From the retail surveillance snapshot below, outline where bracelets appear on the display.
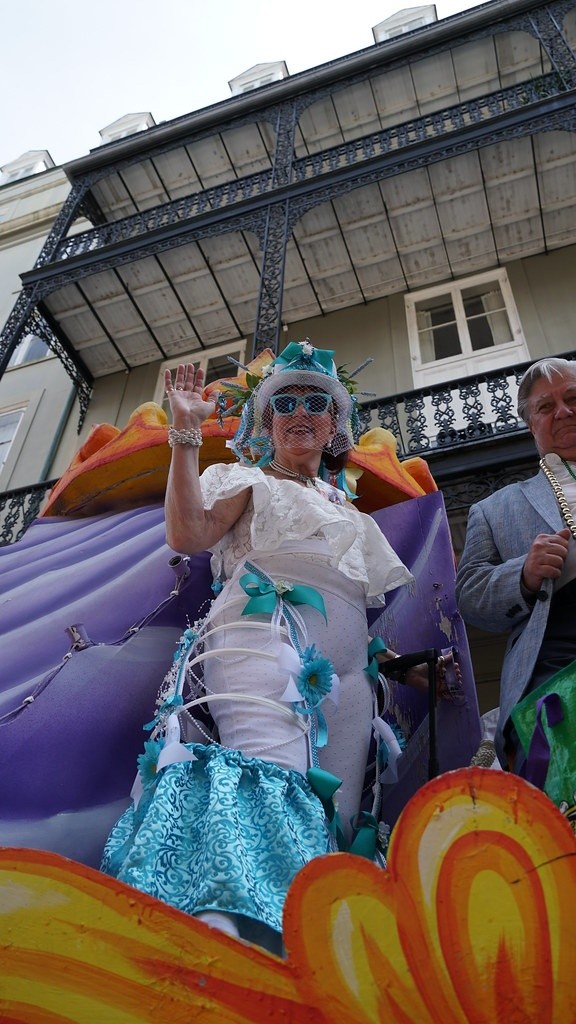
[390,669,408,685]
[168,427,203,447]
[395,655,401,658]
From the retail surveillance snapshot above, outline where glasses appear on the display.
[269,393,333,417]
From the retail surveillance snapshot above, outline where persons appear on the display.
[98,338,464,953]
[454,357,576,788]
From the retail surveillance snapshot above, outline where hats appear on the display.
[216,337,376,466]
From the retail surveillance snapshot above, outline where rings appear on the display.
[166,388,174,393]
[177,387,184,390]
[439,656,444,661]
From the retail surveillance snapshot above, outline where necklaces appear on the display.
[269,460,315,485]
[539,458,576,538]
[559,456,576,480]
